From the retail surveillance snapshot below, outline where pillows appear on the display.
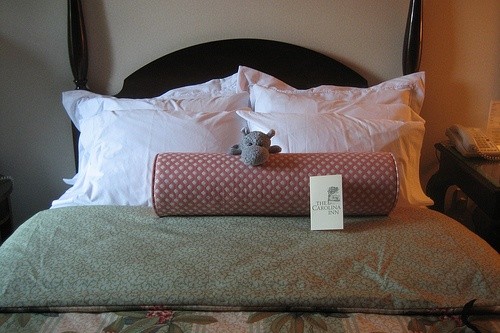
[240,64,426,116]
[153,151,401,218]
[239,107,436,212]
[54,107,247,206]
[61,74,250,188]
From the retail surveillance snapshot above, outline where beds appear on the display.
[0,1,499,333]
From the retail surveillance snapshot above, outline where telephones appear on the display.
[446,124,500,159]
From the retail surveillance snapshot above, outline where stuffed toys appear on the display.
[230,127,282,165]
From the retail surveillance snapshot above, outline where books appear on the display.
[309,174,344,230]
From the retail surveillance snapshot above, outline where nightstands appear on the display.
[426,139,500,252]
